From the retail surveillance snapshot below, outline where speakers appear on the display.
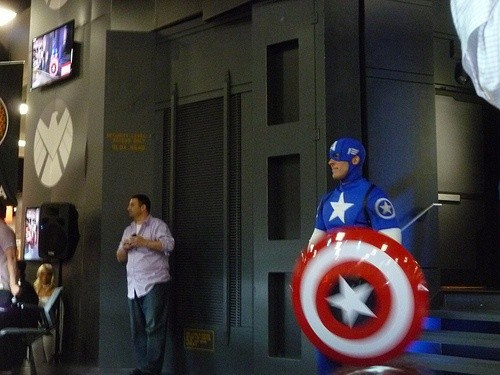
[38,201,79,262]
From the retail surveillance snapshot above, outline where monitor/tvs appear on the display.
[30,19,80,91]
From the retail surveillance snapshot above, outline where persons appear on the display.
[116,194,174,375]
[0,196,22,375]
[31,264,63,375]
[303,136,401,375]
[15,259,39,307]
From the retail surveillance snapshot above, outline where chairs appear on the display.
[0,287,63,375]
